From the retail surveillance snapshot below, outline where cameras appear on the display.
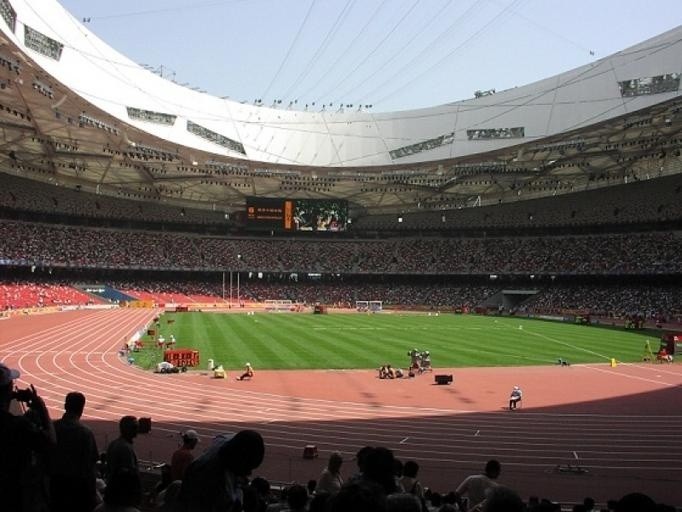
[15,389,31,402]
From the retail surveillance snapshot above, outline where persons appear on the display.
[0,232,682,326]
[1,171,246,226]
[0,331,682,512]
[292,200,346,231]
[350,172,681,232]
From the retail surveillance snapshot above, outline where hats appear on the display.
[0,364,19,385]
[183,430,200,441]
